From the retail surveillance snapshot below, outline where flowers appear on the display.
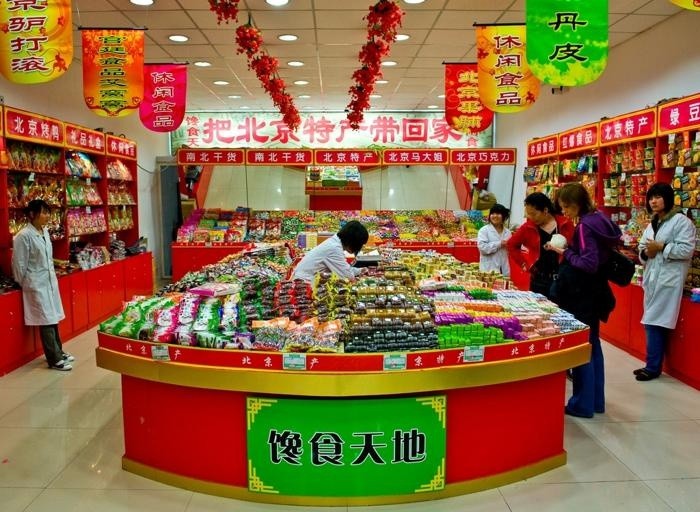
[350,64,384,94]
[264,73,287,96]
[251,48,279,77]
[362,0,406,44]
[279,102,302,133]
[235,12,264,55]
[347,110,364,131]
[271,89,294,106]
[208,0,240,25]
[348,85,369,102]
[346,99,370,113]
[358,35,390,73]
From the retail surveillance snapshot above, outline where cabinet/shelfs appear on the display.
[1,253,152,375]
[3,106,67,275]
[507,250,699,390]
[450,165,491,209]
[657,93,699,289]
[284,165,388,210]
[172,210,490,283]
[179,165,215,210]
[105,133,139,259]
[68,121,107,265]
[524,133,558,222]
[599,105,657,267]
[95,243,590,507]
[558,121,599,209]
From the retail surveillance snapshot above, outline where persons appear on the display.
[291,221,368,279]
[477,205,512,277]
[633,182,697,382]
[12,200,75,372]
[505,192,575,298]
[550,183,622,419]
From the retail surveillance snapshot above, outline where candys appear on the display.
[436,209,517,243]
[315,210,435,244]
[176,207,248,243]
[244,209,314,243]
[99,245,591,355]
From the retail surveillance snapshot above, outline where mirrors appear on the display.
[381,164,448,209]
[445,165,515,210]
[245,165,315,210]
[178,165,247,219]
[314,165,381,210]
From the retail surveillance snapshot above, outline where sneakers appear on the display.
[48,360,72,371]
[636,369,659,381]
[634,367,647,375]
[44,353,74,361]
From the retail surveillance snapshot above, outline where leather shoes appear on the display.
[565,405,593,418]
